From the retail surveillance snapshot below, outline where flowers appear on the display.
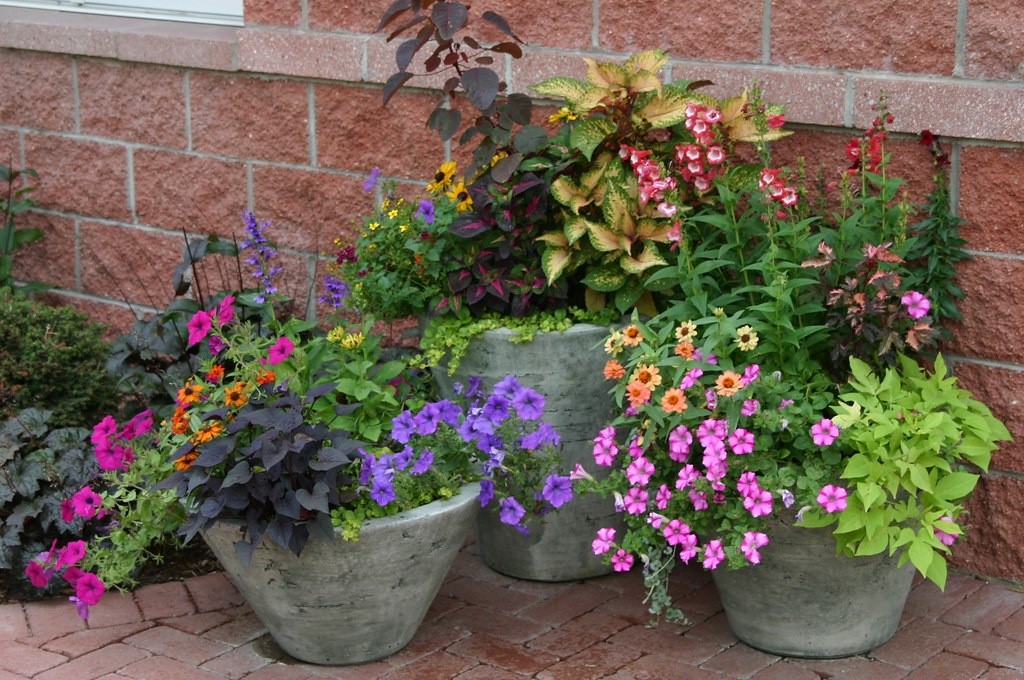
[313,0,786,380]
[28,213,572,630]
[567,83,1013,637]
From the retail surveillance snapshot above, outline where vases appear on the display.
[193,481,489,667]
[417,314,643,583]
[701,474,927,659]
